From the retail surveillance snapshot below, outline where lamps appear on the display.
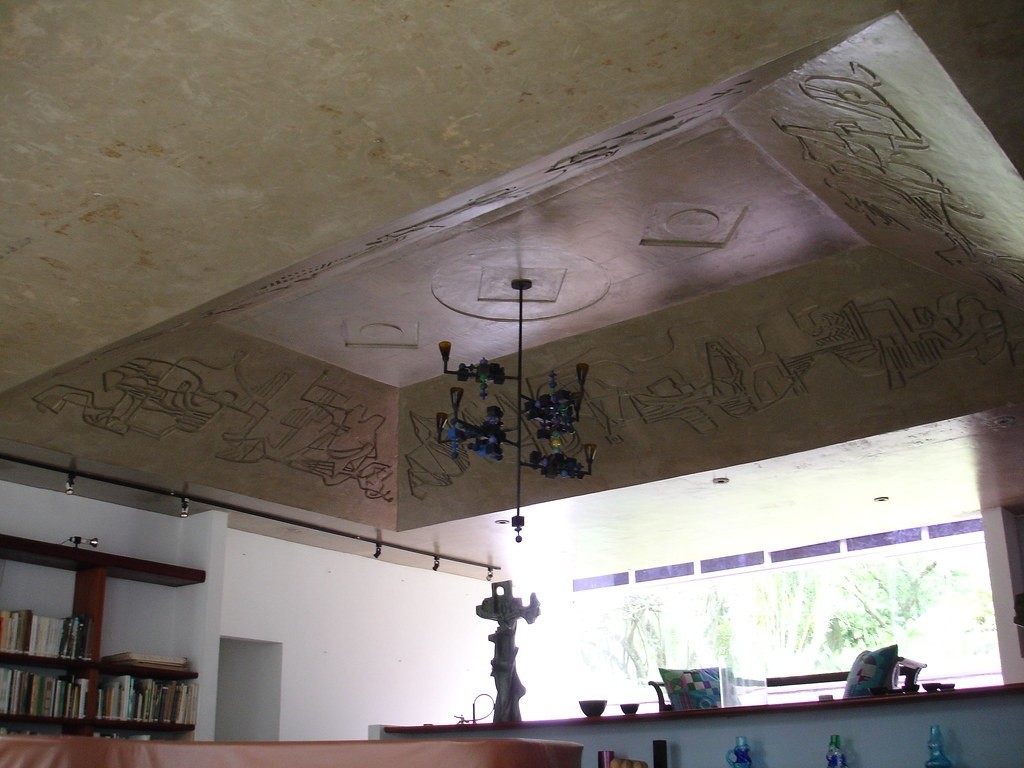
[65,474,75,494]
[486,567,494,581]
[435,279,598,545]
[68,536,99,547]
[432,555,439,570]
[373,543,382,558]
[181,496,189,517]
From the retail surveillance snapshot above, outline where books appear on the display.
[101,651,189,667]
[0,666,199,741]
[0,609,84,658]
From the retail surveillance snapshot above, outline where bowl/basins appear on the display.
[579,700,607,717]
[620,704,639,714]
[939,684,955,690]
[922,683,940,692]
[868,687,888,696]
[901,685,919,693]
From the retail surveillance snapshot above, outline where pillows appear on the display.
[659,667,721,712]
[843,644,899,698]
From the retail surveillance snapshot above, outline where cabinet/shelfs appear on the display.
[0,534,206,740]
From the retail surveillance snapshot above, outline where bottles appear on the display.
[725,736,752,768]
[924,725,953,768]
[826,735,848,768]
